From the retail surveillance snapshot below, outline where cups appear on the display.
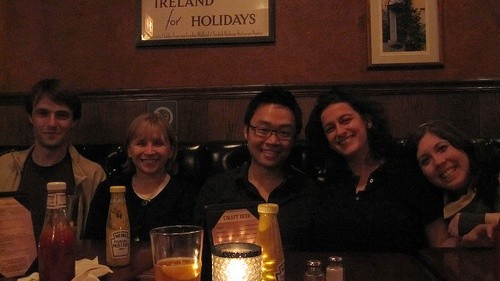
[211,241,263,281]
[149,225,204,281]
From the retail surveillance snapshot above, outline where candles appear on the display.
[211,242,262,281]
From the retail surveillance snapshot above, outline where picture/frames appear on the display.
[134,0,276,48]
[366,0,446,70]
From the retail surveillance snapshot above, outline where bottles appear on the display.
[37,181,76,281]
[326,255,345,281]
[254,203,286,281]
[303,259,325,281]
[105,185,131,267]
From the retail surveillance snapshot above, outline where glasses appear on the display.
[248,124,296,140]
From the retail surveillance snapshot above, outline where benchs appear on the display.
[0,137,500,249]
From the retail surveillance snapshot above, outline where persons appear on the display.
[402,120,500,248]
[192,89,324,252]
[304,86,428,251]
[82,112,196,242]
[0,78,106,240]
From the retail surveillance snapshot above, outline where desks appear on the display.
[35,239,500,281]
